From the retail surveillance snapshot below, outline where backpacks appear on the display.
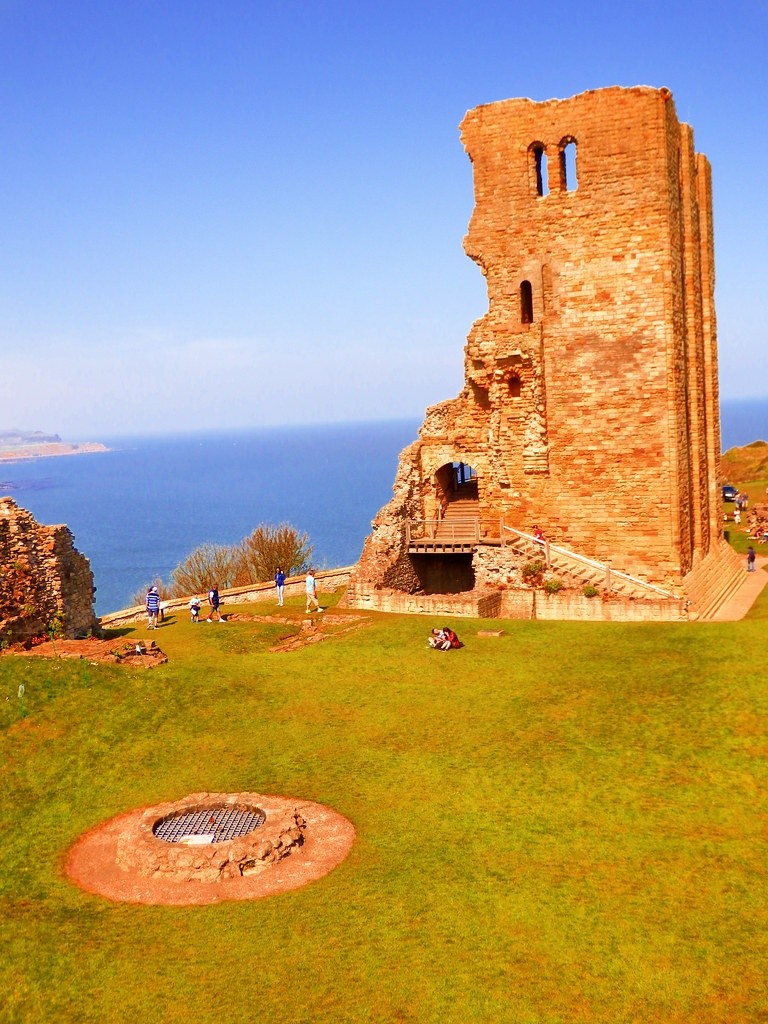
[208,590,213,605]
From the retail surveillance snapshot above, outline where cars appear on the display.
[722,485,740,502]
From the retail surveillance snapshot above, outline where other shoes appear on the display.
[306,609,311,613]
[317,608,323,612]
[438,648,448,652]
[196,621,201,623]
[277,603,283,606]
[219,619,226,622]
[191,617,194,622]
[207,619,212,622]
[147,623,151,630]
[154,626,159,630]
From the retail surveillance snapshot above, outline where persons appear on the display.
[734,490,748,511]
[206,584,226,622]
[305,570,323,613]
[146,586,160,630]
[532,525,543,556]
[758,531,768,544]
[428,627,460,653]
[747,546,754,572]
[734,507,741,528]
[274,567,286,606]
[757,525,764,538]
[190,592,201,623]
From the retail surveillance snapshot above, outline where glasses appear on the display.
[314,573,315,574]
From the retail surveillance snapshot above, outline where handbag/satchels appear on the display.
[435,640,446,649]
[750,558,754,562]
[191,605,197,615]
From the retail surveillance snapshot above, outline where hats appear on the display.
[153,587,157,591]
